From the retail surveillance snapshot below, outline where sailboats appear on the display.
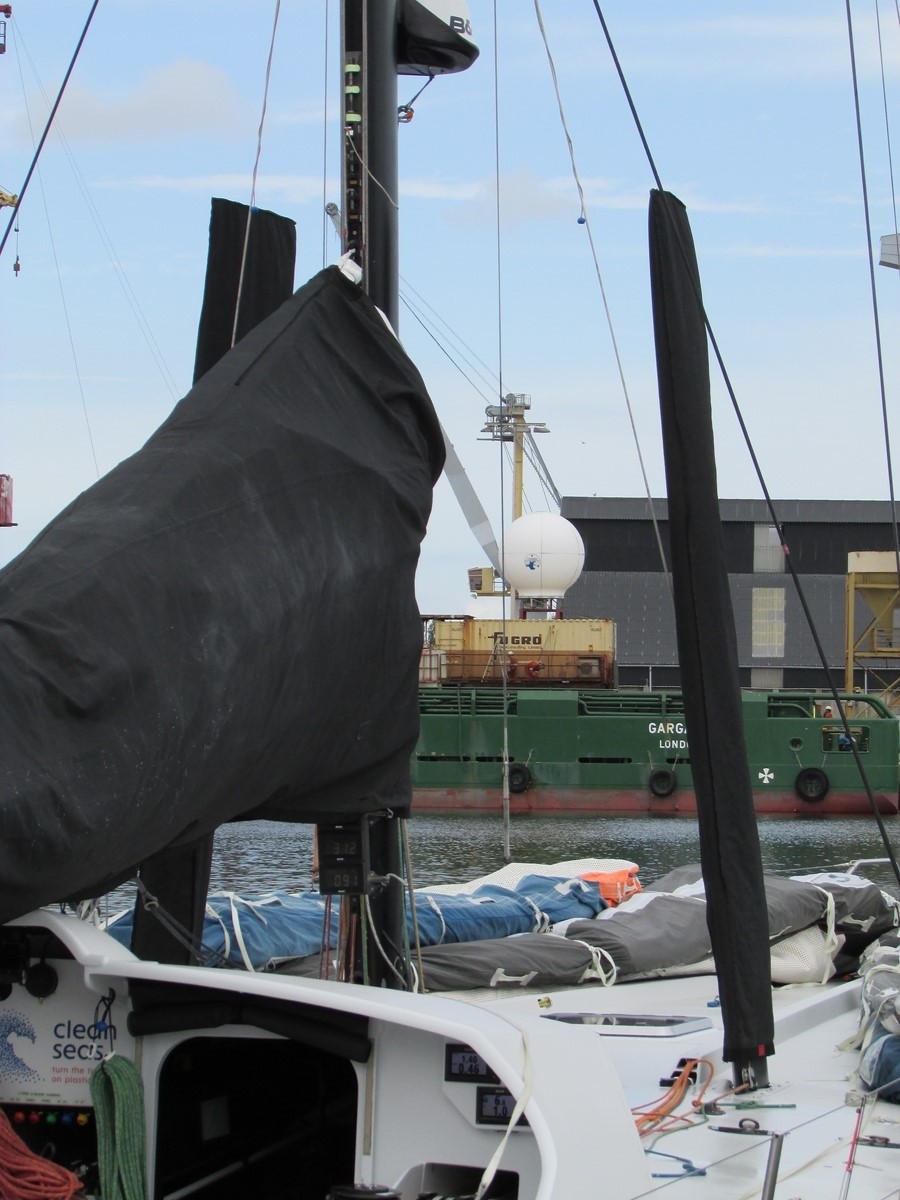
[1,1,900,1200]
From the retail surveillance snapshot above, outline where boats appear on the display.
[406,683,900,817]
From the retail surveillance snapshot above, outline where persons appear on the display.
[823,706,833,730]
[506,651,543,685]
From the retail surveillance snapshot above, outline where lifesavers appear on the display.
[648,768,676,796]
[503,763,531,793]
[795,768,829,802]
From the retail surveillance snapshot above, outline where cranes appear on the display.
[325,204,561,620]
